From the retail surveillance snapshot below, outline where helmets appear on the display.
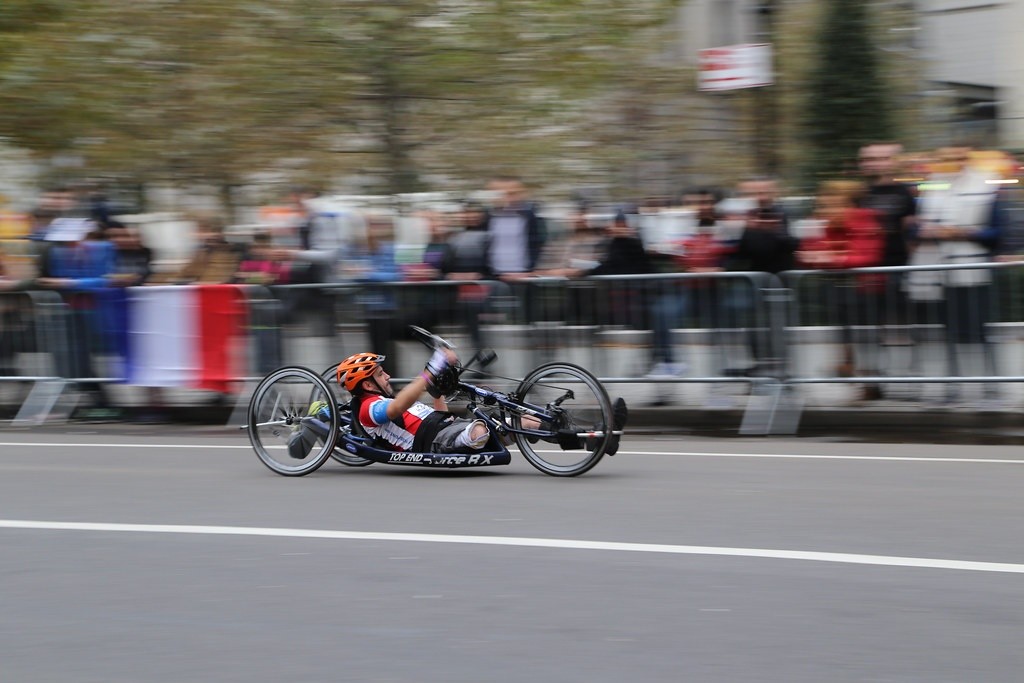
[336,352,386,395]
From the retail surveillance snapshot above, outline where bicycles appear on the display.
[246,324,615,478]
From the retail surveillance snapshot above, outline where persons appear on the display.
[338,348,559,455]
[0,144,1024,425]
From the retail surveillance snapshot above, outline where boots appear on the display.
[586,397,627,456]
